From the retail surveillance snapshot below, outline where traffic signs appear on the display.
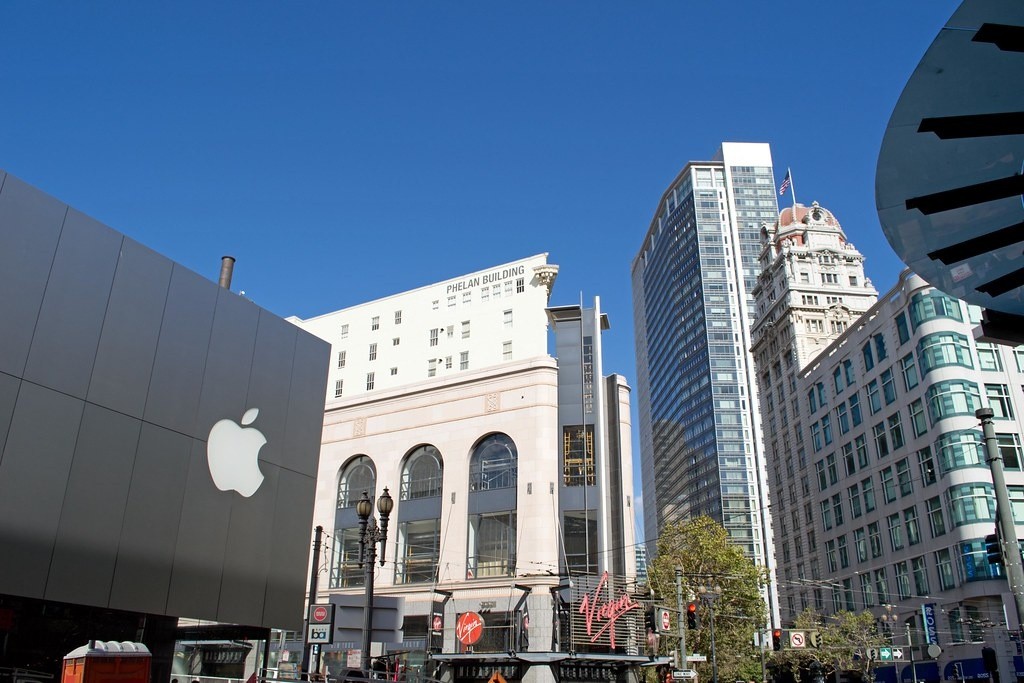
[672,669,698,680]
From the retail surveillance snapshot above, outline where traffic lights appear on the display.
[951,664,960,680]
[774,630,782,653]
[645,607,656,631]
[687,604,699,630]
[815,633,822,648]
[668,649,678,669]
[871,649,876,659]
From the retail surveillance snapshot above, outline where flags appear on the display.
[780,171,791,195]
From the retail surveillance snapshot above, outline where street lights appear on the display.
[698,577,722,683]
[355,485,394,679]
[882,607,900,683]
[909,646,920,683]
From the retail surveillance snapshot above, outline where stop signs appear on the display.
[660,612,671,631]
